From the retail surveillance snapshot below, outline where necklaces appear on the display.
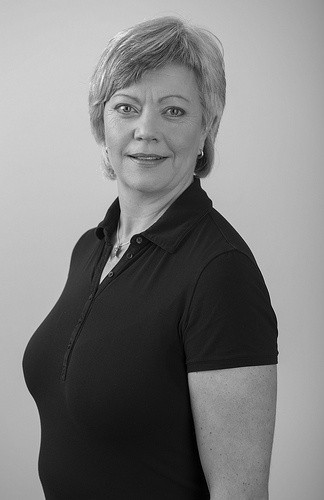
[114,219,130,256]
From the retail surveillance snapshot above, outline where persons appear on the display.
[22,16,280,500]
